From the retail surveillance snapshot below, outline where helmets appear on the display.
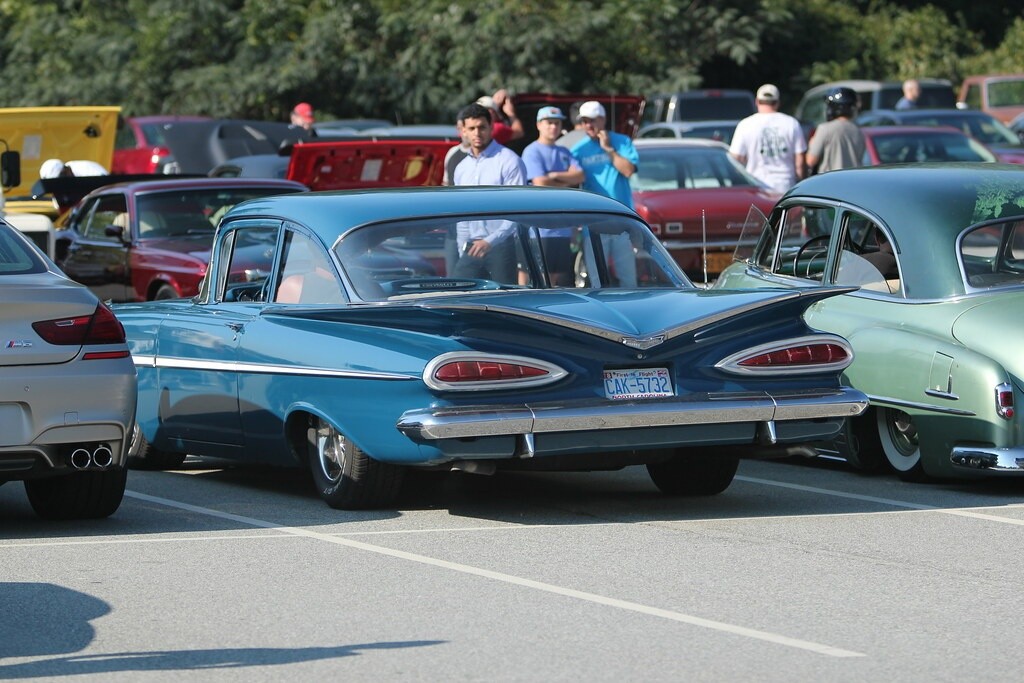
[821,87,857,104]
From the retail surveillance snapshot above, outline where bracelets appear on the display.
[607,146,614,153]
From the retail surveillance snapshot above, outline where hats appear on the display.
[293,102,316,123]
[756,83,781,102]
[536,106,568,122]
[38,157,64,180]
[474,95,507,122]
[575,101,607,121]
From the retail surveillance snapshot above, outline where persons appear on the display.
[520,106,586,290]
[290,100,318,140]
[473,91,524,145]
[554,100,640,288]
[895,79,920,109]
[870,221,894,255]
[804,86,865,176]
[727,84,807,198]
[441,103,527,286]
[39,158,111,218]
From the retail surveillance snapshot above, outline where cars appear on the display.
[51,176,439,308]
[708,158,1023,486]
[95,184,871,514]
[0,214,140,526]
[0,73,1024,282]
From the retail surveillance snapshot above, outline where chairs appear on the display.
[112,210,167,233]
[857,252,898,281]
